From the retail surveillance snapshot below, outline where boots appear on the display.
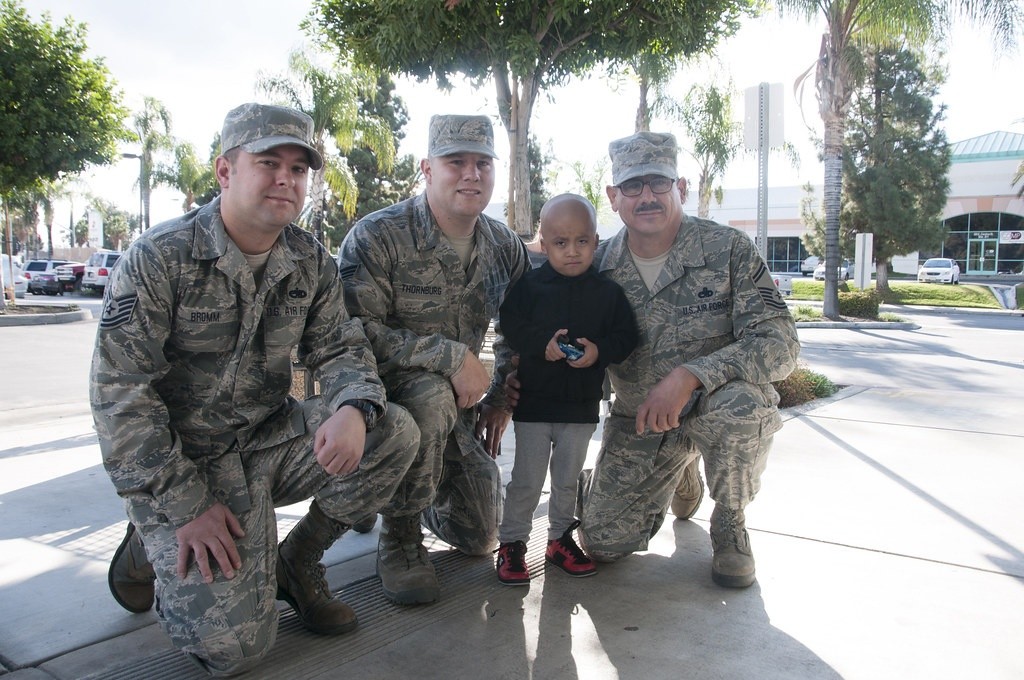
[375,509,442,606]
[108,520,158,613]
[273,501,357,632]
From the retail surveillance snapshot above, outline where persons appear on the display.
[503,131,801,587]
[336,114,534,608]
[492,193,639,585]
[88,102,422,680]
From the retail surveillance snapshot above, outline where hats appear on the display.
[220,103,322,171]
[609,131,678,185]
[429,115,498,160]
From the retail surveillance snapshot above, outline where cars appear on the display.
[813,258,854,281]
[31,273,58,296]
[801,255,821,276]
[918,258,960,284]
[2,254,29,297]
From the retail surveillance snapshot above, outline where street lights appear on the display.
[122,153,142,235]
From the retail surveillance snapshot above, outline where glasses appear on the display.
[615,176,680,197]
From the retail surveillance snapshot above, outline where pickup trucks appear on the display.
[771,275,792,298]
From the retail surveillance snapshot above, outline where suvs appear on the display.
[82,251,122,294]
[54,264,86,290]
[20,259,80,292]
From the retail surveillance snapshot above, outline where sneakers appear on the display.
[671,454,703,520]
[493,540,531,586]
[546,519,596,578]
[709,502,755,587]
